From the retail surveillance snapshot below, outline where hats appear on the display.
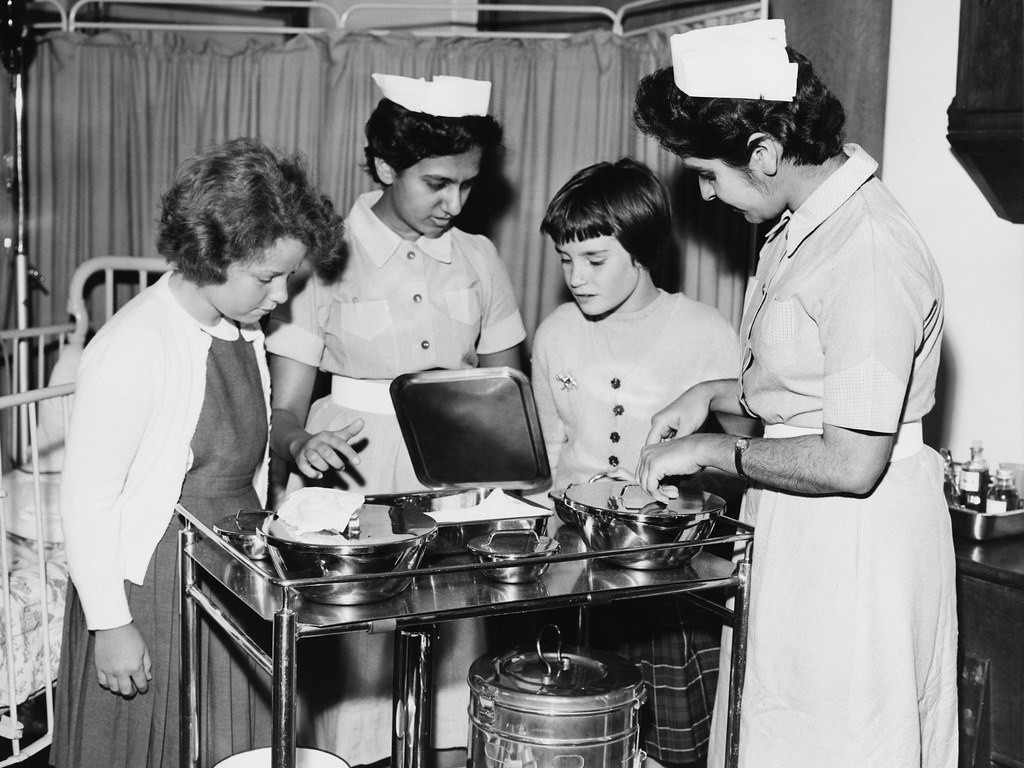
[670,20,798,103]
[373,70,491,118]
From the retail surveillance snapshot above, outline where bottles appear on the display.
[959,447,989,513]
[986,470,1019,513]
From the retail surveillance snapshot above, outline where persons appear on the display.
[270,78,530,768]
[529,153,758,768]
[46,136,347,768]
[632,40,962,768]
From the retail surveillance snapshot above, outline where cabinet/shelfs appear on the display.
[172,488,755,768]
[953,533,1024,768]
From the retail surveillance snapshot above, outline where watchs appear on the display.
[734,433,756,481]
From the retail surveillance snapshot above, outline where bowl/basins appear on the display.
[549,490,586,525]
[565,481,727,568]
[467,529,560,583]
[256,505,440,602]
[212,747,350,768]
[213,508,277,560]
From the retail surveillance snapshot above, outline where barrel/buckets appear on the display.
[469,623,646,768]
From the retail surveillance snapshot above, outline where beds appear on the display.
[0,254,180,743]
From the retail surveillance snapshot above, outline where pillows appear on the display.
[23,344,85,473]
